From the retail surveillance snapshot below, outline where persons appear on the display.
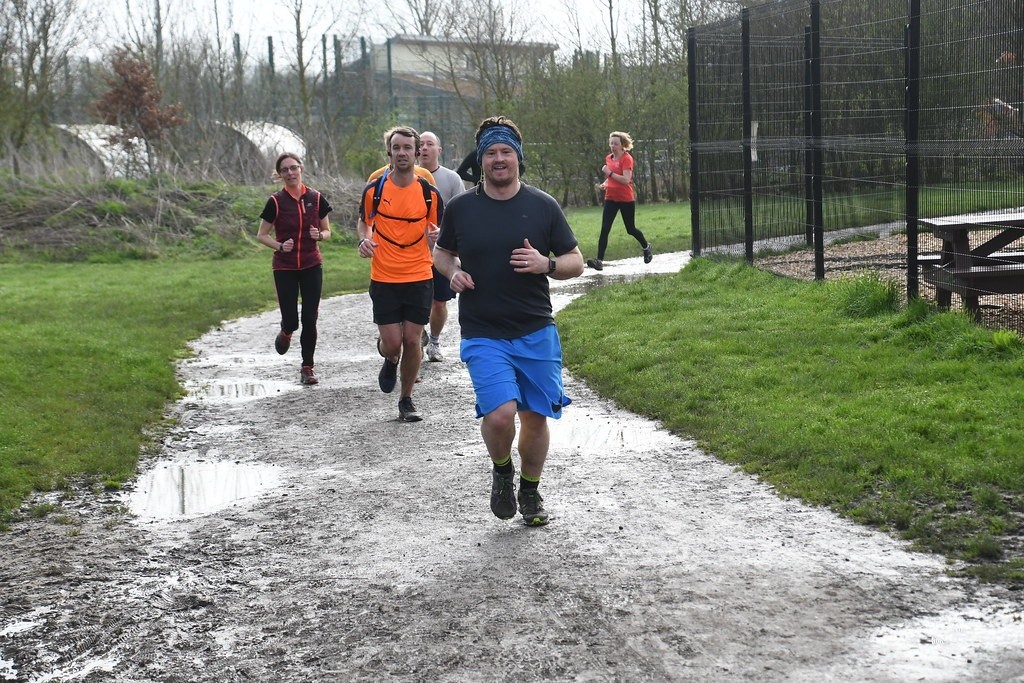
[457,150,525,186]
[367,127,436,382]
[358,127,445,421]
[587,131,652,271]
[418,131,466,362]
[432,115,584,525]
[257,152,333,384]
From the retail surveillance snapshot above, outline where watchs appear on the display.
[547,258,556,274]
[318,234,323,241]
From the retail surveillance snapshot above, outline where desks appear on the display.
[917,213,1024,322]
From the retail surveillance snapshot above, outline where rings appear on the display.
[526,262,527,266]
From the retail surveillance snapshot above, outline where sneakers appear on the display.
[644,244,652,264]
[274,330,292,355]
[491,464,517,520]
[587,259,603,271]
[518,489,548,526]
[426,342,443,362]
[300,366,318,384]
[421,330,429,360]
[398,397,422,421]
[379,355,400,393]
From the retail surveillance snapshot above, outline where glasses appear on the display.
[279,165,301,173]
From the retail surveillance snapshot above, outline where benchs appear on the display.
[916,252,1024,298]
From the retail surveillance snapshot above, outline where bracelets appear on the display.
[280,244,283,251]
[358,240,364,247]
[609,172,613,177]
